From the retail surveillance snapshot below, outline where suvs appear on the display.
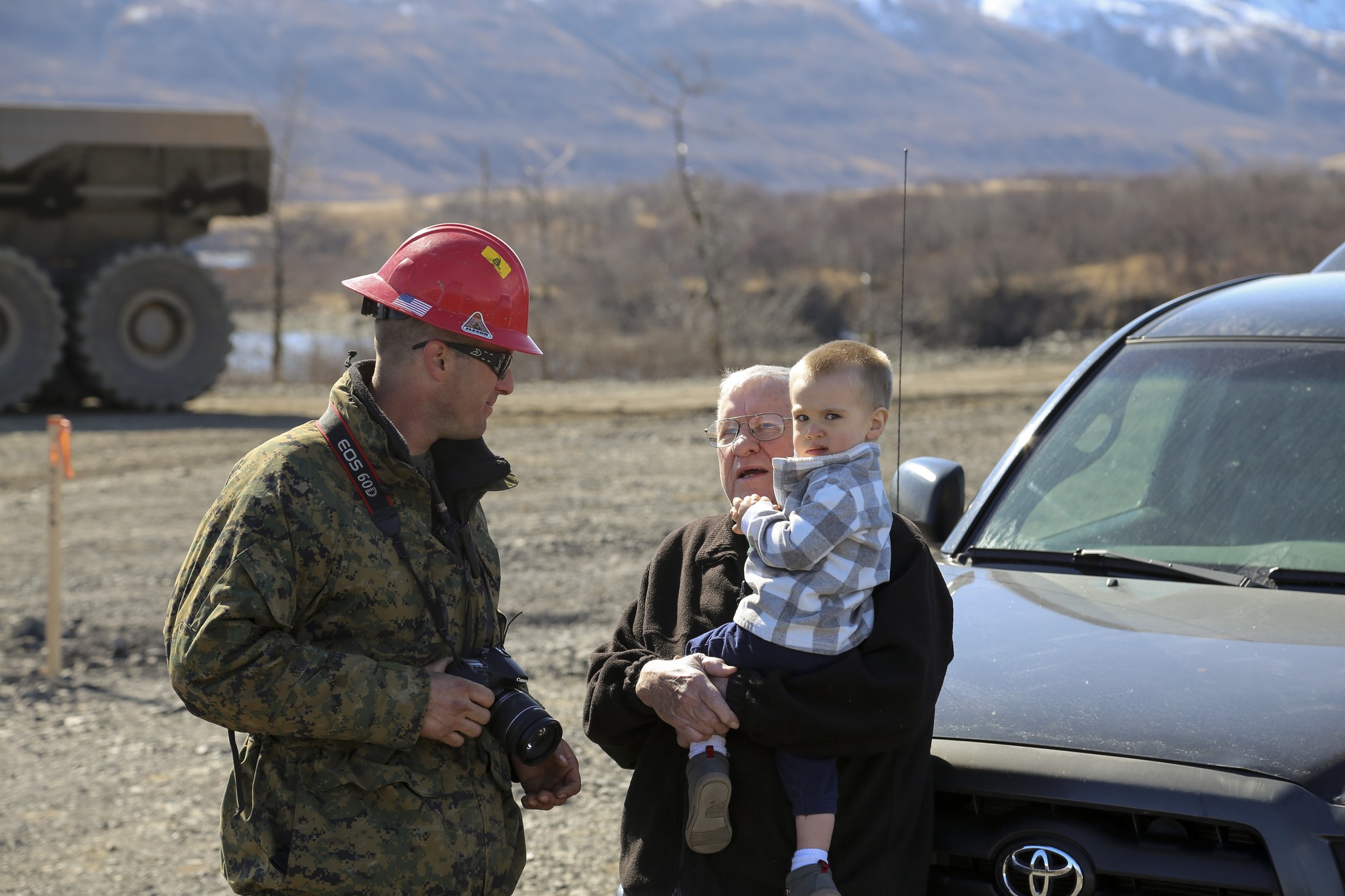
[890,237,1345,896]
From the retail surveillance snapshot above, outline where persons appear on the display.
[582,365,954,896]
[164,223,582,896]
[683,339,890,896]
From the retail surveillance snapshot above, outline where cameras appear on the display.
[444,647,563,767]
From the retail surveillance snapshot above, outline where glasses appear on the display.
[705,412,792,448]
[413,338,513,380]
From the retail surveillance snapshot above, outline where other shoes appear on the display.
[785,858,840,896]
[683,742,731,853]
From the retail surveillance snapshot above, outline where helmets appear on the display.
[341,223,544,355]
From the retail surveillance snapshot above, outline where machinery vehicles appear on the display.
[0,96,272,416]
